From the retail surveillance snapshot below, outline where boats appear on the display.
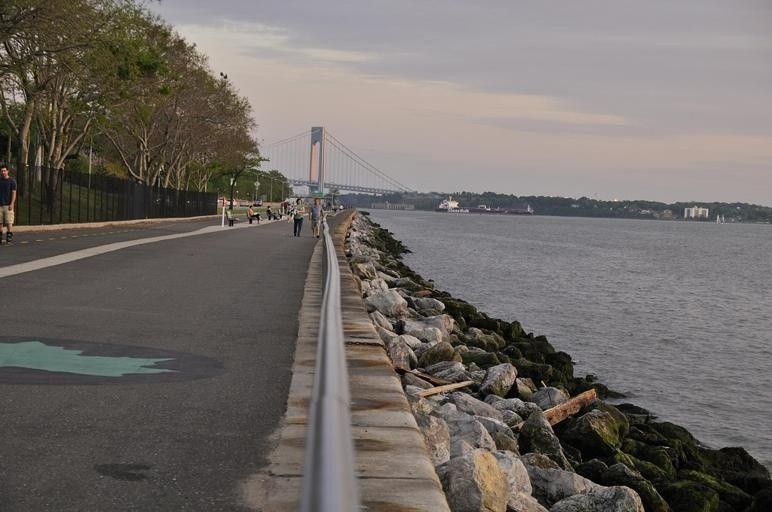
[431,194,535,215]
[448,208,470,214]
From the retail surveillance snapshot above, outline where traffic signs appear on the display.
[311,192,324,198]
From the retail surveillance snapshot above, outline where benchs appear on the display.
[225,209,286,227]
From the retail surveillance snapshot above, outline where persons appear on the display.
[0,165,19,244]
[249,205,264,224]
[308,197,325,238]
[266,205,280,220]
[280,200,296,224]
[292,197,306,237]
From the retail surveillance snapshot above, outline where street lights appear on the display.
[247,170,304,201]
[332,193,335,210]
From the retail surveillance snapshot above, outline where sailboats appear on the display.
[722,212,727,224]
[716,213,722,224]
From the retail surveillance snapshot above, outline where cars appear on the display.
[150,197,263,209]
